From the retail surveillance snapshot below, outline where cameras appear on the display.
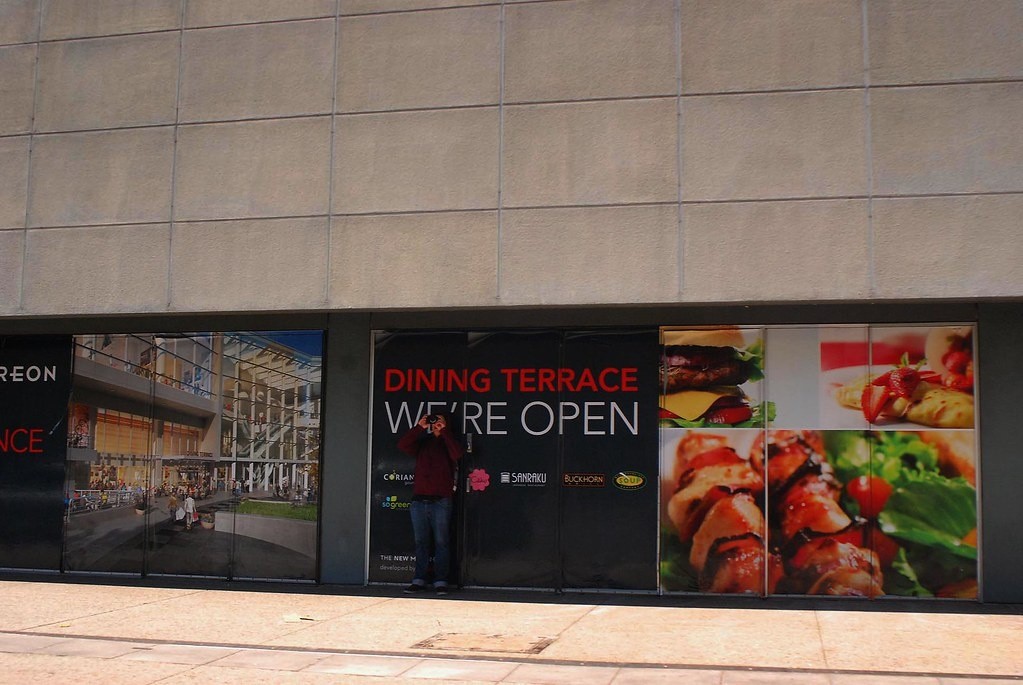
[425,415,438,424]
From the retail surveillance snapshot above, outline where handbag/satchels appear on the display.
[192,511,199,523]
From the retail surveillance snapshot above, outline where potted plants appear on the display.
[135,500,145,515]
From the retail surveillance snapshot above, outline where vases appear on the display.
[201,521,215,530]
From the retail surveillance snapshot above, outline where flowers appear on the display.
[201,513,214,522]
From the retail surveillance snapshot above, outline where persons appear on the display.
[120,483,142,504]
[161,476,210,499]
[183,496,197,529]
[72,490,79,498]
[168,492,178,525]
[397,410,468,596]
[211,478,245,493]
[82,489,107,510]
[275,482,304,499]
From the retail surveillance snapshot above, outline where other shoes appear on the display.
[404,584,426,593]
[437,586,447,596]
